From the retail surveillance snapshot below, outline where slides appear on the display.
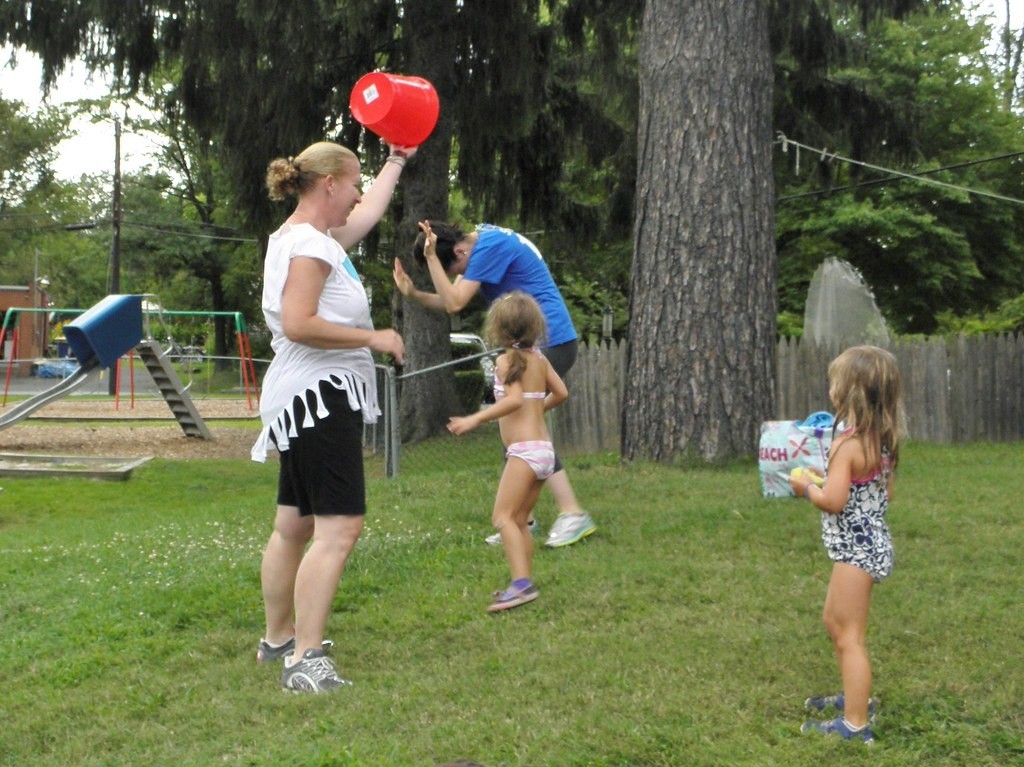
[0,366,101,431]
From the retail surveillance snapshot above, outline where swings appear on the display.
[37,313,58,392]
[149,316,171,396]
[76,363,99,394]
[188,317,210,401]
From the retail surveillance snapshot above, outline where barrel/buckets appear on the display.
[349,72,441,147]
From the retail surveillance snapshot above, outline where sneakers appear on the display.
[485,520,537,547]
[282,640,361,696]
[544,511,598,547]
[800,715,874,747]
[486,584,539,612]
[257,637,295,666]
[805,691,876,722]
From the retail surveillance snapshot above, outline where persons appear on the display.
[391,219,600,549]
[447,289,572,615]
[790,344,910,751]
[252,142,417,695]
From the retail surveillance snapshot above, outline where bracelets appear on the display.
[803,483,815,498]
[386,150,408,167]
